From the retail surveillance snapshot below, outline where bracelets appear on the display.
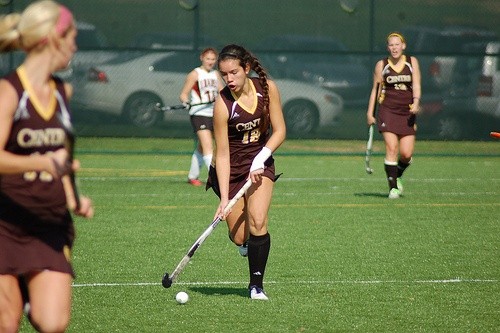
[413,96,420,99]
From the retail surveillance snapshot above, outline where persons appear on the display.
[367,31,421,199]
[204,45,286,301]
[0,0,92,333]
[179,48,224,187]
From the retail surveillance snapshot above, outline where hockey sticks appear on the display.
[161,177,253,288]
[54,111,81,209]
[489,132,500,138]
[365,82,380,174]
[154,99,215,112]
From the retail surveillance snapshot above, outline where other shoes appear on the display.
[188,179,202,186]
[396,178,403,191]
[388,188,399,199]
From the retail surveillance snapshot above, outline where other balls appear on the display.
[175,291,188,304]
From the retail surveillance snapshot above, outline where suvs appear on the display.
[0,20,108,81]
[404,24,499,139]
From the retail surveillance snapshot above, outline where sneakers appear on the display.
[238,242,248,256]
[249,285,269,300]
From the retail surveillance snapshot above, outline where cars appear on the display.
[250,35,368,106]
[71,43,344,136]
